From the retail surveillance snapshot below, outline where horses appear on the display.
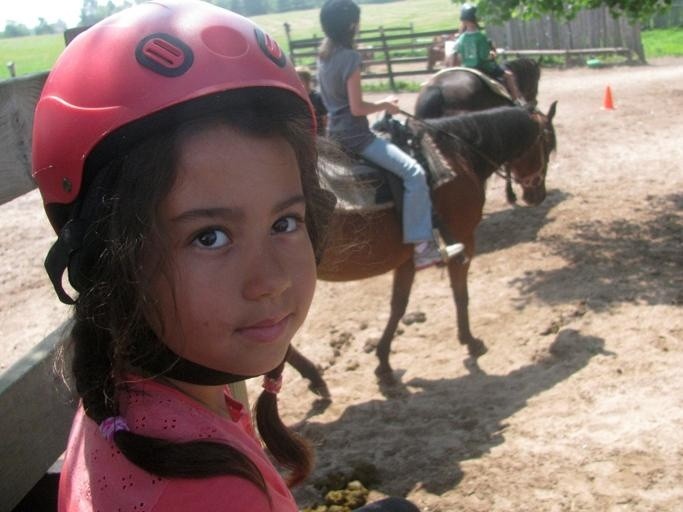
[283,99,559,399]
[414,51,545,204]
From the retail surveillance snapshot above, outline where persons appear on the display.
[293,64,328,140]
[448,3,525,108]
[27,2,422,511]
[316,0,466,273]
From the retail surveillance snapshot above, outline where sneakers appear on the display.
[412,241,465,268]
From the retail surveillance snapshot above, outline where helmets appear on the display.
[30,1,318,237]
[460,4,476,23]
[321,0,359,40]
